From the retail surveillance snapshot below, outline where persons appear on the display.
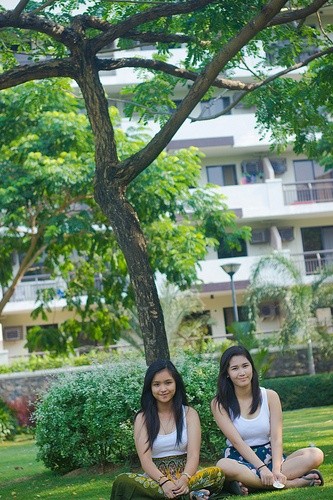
[210,345,325,496]
[111,360,226,500]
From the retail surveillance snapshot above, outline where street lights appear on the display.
[220,262,242,322]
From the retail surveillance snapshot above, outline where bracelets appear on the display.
[157,475,171,487]
[258,464,267,471]
[182,472,191,479]
